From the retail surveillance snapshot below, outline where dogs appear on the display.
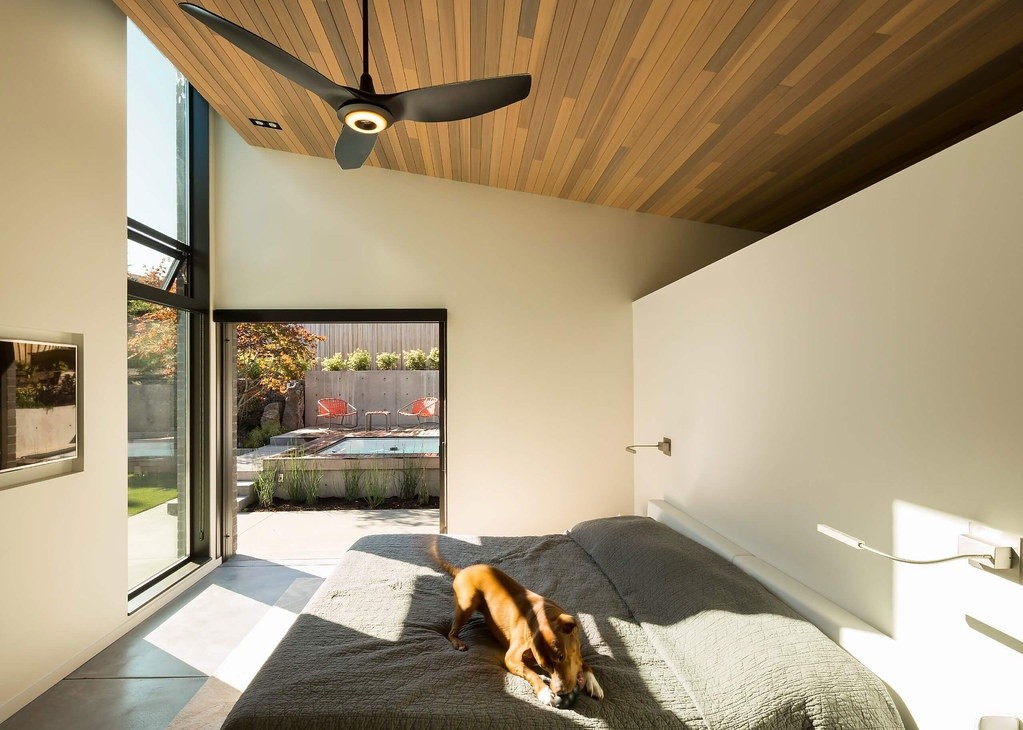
[427,533,605,708]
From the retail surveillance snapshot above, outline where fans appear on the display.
[178,0,532,171]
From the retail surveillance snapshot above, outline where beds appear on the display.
[216,498,1023,730]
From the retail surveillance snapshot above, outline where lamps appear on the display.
[815,524,1012,571]
[626,437,671,456]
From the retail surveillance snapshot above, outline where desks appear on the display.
[365,411,391,432]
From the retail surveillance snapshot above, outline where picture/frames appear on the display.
[1,325,84,491]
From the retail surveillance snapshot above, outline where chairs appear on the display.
[315,397,358,430]
[396,396,440,430]
